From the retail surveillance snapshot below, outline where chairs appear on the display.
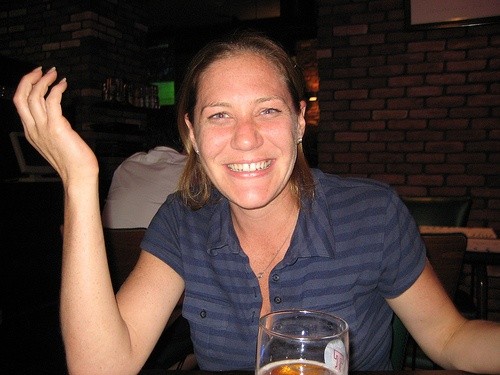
[104,228,193,375]
[390,196,473,370]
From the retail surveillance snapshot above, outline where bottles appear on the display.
[0,87,9,102]
[102,80,158,109]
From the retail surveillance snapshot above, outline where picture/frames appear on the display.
[403,0,500,31]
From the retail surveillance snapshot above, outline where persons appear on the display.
[102,116,190,229]
[13,36,500,375]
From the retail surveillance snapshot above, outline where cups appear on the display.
[254,309,349,375]
